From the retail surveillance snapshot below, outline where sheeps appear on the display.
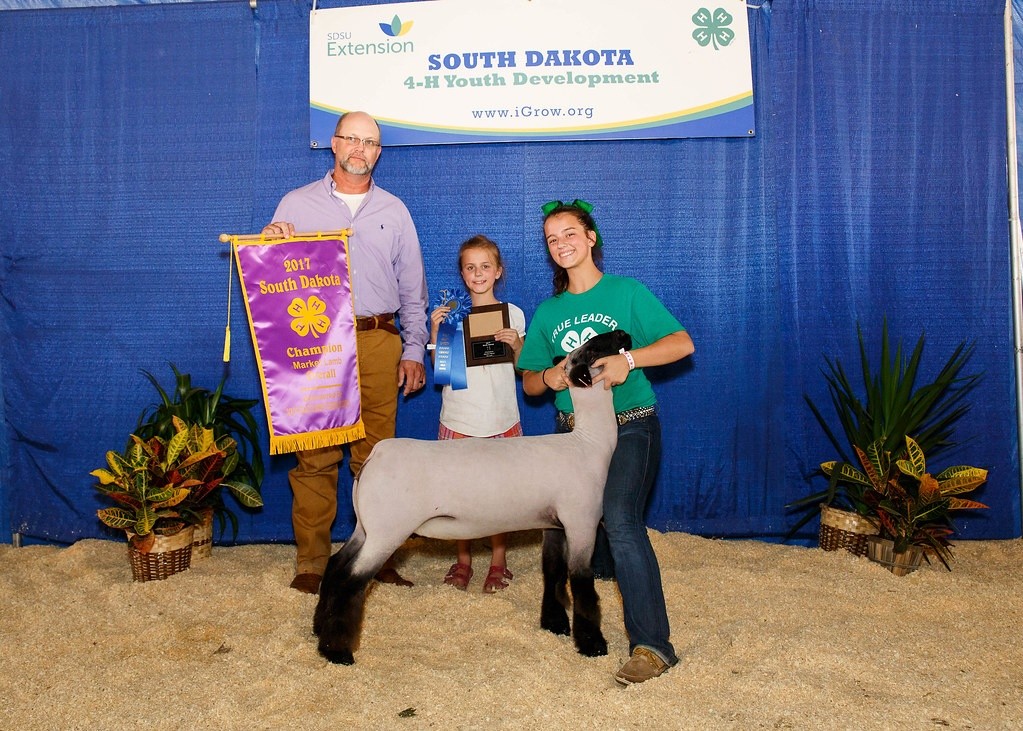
[312,330,634,665]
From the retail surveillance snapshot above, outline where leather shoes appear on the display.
[374,567,414,586]
[289,573,322,594]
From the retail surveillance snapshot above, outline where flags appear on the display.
[219,228,368,456]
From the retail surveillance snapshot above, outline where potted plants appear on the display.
[819,435,990,577]
[89,413,237,583]
[127,357,265,563]
[782,309,988,558]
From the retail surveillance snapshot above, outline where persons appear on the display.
[260,110,431,595]
[514,199,696,687]
[428,233,527,594]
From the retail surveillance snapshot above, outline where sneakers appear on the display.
[614,647,668,686]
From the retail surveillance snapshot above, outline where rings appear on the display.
[419,381,423,383]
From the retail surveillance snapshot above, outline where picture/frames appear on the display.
[463,301,514,367]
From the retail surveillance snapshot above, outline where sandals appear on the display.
[444,563,474,591]
[483,565,512,594]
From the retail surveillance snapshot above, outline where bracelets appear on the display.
[426,343,436,350]
[618,348,635,370]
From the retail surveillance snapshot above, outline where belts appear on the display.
[356,312,400,335]
[555,405,656,431]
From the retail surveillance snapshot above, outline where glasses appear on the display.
[335,135,380,146]
